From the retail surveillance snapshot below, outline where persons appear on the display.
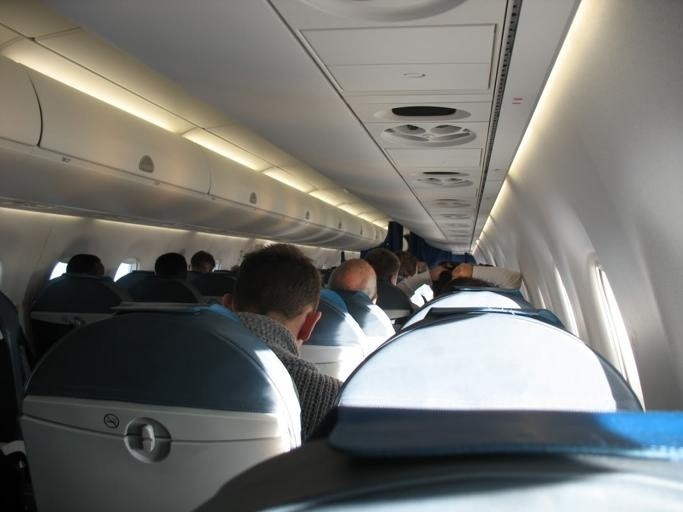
[396,251,523,298]
[67,253,102,275]
[221,243,341,440]
[328,257,396,337]
[191,251,214,273]
[365,247,412,325]
[156,253,187,281]
[396,251,418,278]
